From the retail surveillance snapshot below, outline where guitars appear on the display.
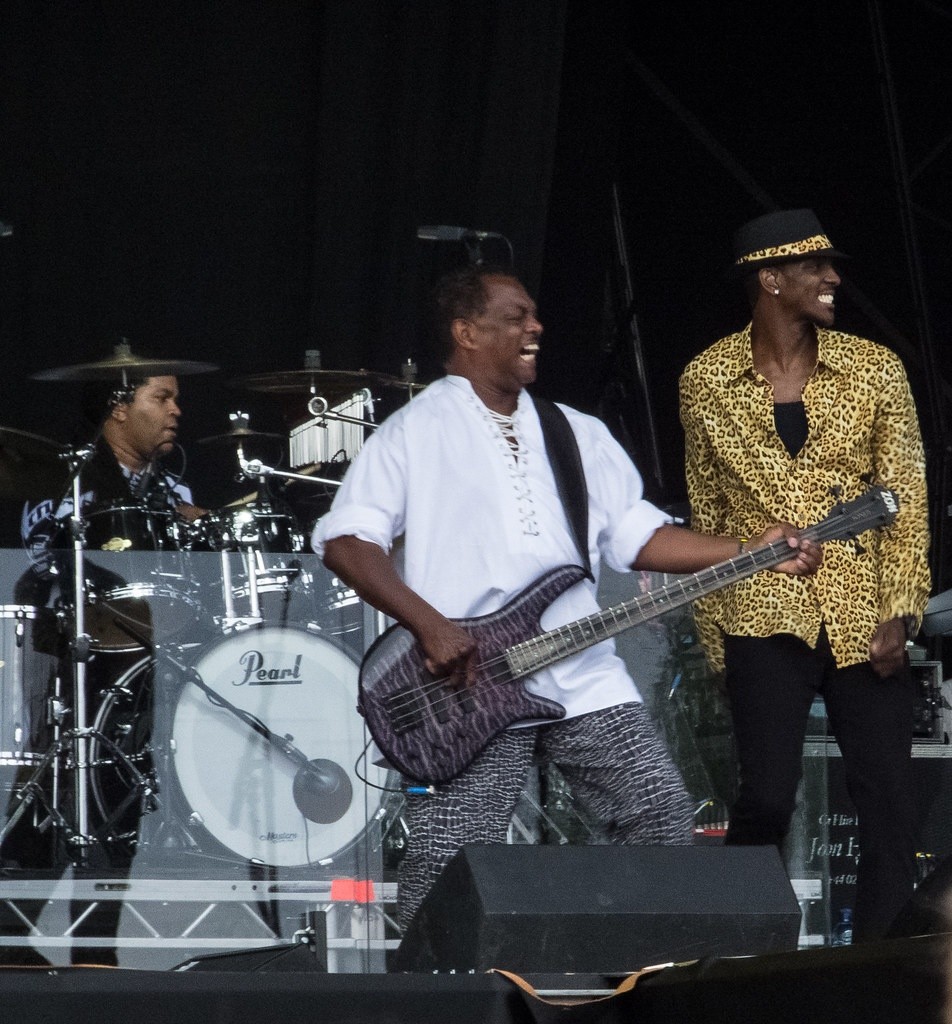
[356,485,900,786]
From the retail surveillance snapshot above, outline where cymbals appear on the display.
[238,369,427,393]
[193,428,283,447]
[0,423,71,504]
[29,355,220,382]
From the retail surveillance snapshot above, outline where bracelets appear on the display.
[738,540,744,555]
[903,615,917,637]
[905,640,914,650]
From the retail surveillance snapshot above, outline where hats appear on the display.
[731,208,852,267]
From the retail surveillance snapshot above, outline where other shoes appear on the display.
[0,946,52,969]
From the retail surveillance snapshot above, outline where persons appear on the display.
[309,271,822,944]
[680,208,952,946]
[1,375,206,967]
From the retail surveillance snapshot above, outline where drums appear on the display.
[0,603,60,797]
[190,501,364,635]
[49,504,197,653]
[89,621,398,868]
